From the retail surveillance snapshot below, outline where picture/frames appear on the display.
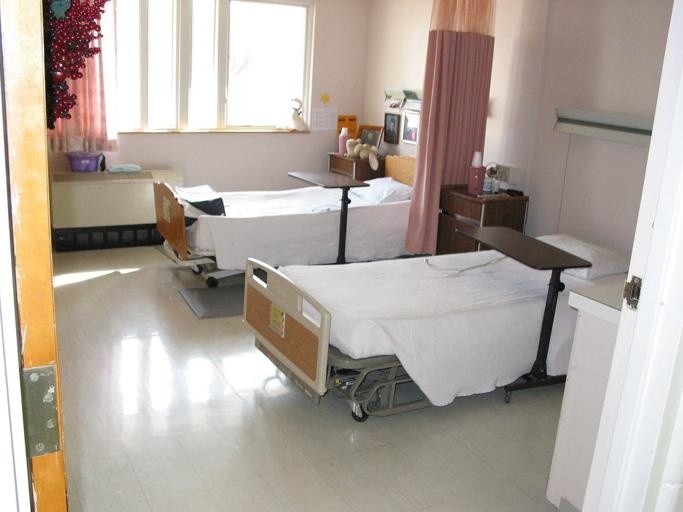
[383,113,399,144]
[401,111,420,145]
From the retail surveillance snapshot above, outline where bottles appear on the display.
[338,127,348,154]
[467,151,500,196]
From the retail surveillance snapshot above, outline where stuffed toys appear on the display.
[344,137,379,171]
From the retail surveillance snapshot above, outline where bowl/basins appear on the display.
[65,151,101,172]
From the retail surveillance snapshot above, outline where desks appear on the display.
[456,225,592,394]
[288,170,368,262]
[546,280,624,511]
[51,168,185,252]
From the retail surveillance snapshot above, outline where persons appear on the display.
[387,119,397,138]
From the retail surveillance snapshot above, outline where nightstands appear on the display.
[329,151,383,183]
[436,184,528,256]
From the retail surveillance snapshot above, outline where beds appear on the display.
[241,233,627,423]
[153,155,416,287]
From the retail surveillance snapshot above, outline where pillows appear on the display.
[349,177,412,204]
[534,233,628,279]
